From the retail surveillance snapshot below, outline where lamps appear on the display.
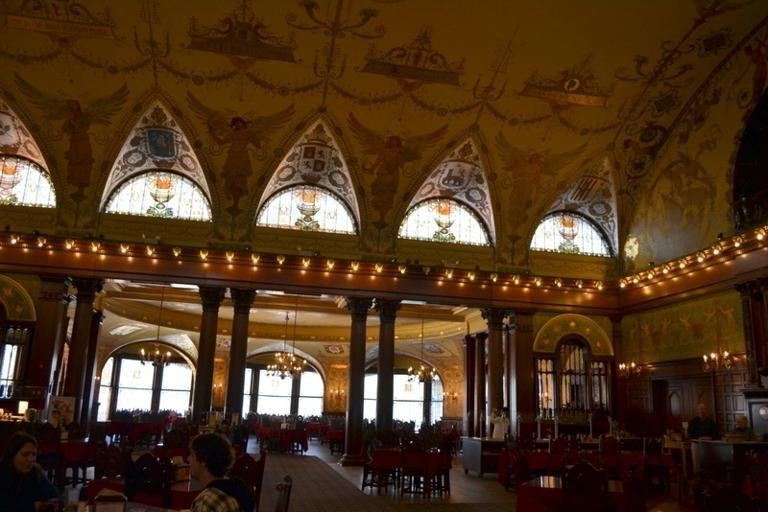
[699,292,747,374]
[614,305,656,379]
[406,312,440,384]
[442,390,459,402]
[138,279,175,369]
[264,295,311,382]
[327,389,346,401]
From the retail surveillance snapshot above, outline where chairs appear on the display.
[1,406,346,510]
[360,408,766,511]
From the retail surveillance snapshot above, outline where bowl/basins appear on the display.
[721,431,745,442]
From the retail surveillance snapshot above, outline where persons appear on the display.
[0,435,57,512]
[687,404,718,440]
[490,407,509,440]
[731,415,755,461]
[182,432,255,512]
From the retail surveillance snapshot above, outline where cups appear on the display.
[77,501,89,512]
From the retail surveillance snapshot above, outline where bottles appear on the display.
[52,404,62,426]
